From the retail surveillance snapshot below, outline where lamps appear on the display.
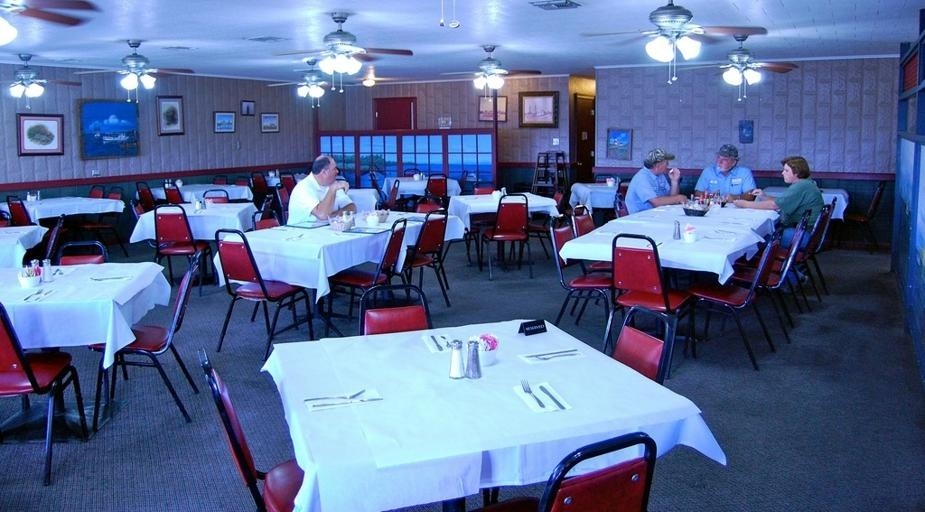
[0,54,82,87]
[8,79,46,109]
[0,9,18,48]
[120,72,157,105]
[0,1,101,27]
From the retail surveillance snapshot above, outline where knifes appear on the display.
[526,348,577,359]
[312,396,385,409]
[538,385,565,410]
[431,335,443,352]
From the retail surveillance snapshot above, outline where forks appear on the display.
[521,381,547,409]
[304,388,366,404]
[536,352,576,361]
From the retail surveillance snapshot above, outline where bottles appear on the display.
[466,341,482,379]
[501,186,507,199]
[672,220,681,240]
[201,197,206,209]
[31,259,40,268]
[447,339,465,379]
[348,210,356,228]
[42,259,55,283]
[420,173,423,181]
[342,211,349,224]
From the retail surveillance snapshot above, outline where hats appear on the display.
[716,143,738,158]
[645,148,675,165]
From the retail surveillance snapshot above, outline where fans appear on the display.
[72,40,195,75]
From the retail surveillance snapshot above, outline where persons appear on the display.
[287,155,356,299]
[733,157,825,293]
[694,144,757,201]
[625,150,687,214]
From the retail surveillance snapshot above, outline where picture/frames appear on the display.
[156,95,185,136]
[260,112,280,134]
[241,100,256,116]
[477,95,508,123]
[17,113,65,157]
[213,110,236,134]
[76,98,141,161]
[606,127,633,161]
[518,91,559,129]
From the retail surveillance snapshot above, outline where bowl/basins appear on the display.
[683,232,697,244]
[16,270,42,288]
[607,181,614,188]
[470,337,500,368]
[682,206,710,216]
[741,194,757,202]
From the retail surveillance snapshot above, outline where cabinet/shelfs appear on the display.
[891,26,924,391]
[531,151,568,195]
